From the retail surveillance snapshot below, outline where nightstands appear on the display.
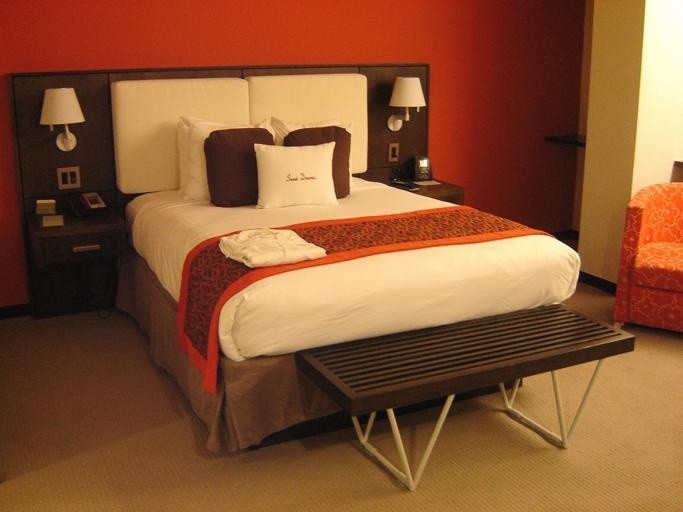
[19,197,129,273]
[380,174,465,213]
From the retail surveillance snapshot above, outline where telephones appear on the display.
[68,191,110,216]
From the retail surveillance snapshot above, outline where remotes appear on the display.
[391,177,417,188]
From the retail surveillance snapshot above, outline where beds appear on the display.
[105,61,588,460]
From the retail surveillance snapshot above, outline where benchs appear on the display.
[291,298,639,493]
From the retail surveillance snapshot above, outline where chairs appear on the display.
[609,171,683,338]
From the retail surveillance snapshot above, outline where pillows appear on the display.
[271,112,356,195]
[172,111,203,200]
[179,117,276,206]
[246,138,339,210]
[196,125,276,208]
[280,125,353,201]
[273,115,346,126]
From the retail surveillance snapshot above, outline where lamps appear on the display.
[382,75,428,136]
[36,86,88,152]
